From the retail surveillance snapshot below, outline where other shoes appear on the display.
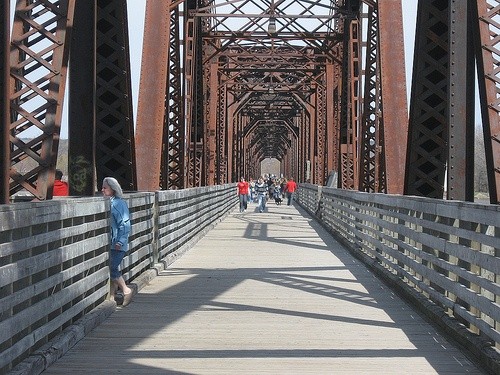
[122,287,134,306]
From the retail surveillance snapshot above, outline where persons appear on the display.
[237,176,250,213]
[101,177,134,307]
[52,170,69,196]
[250,173,297,213]
